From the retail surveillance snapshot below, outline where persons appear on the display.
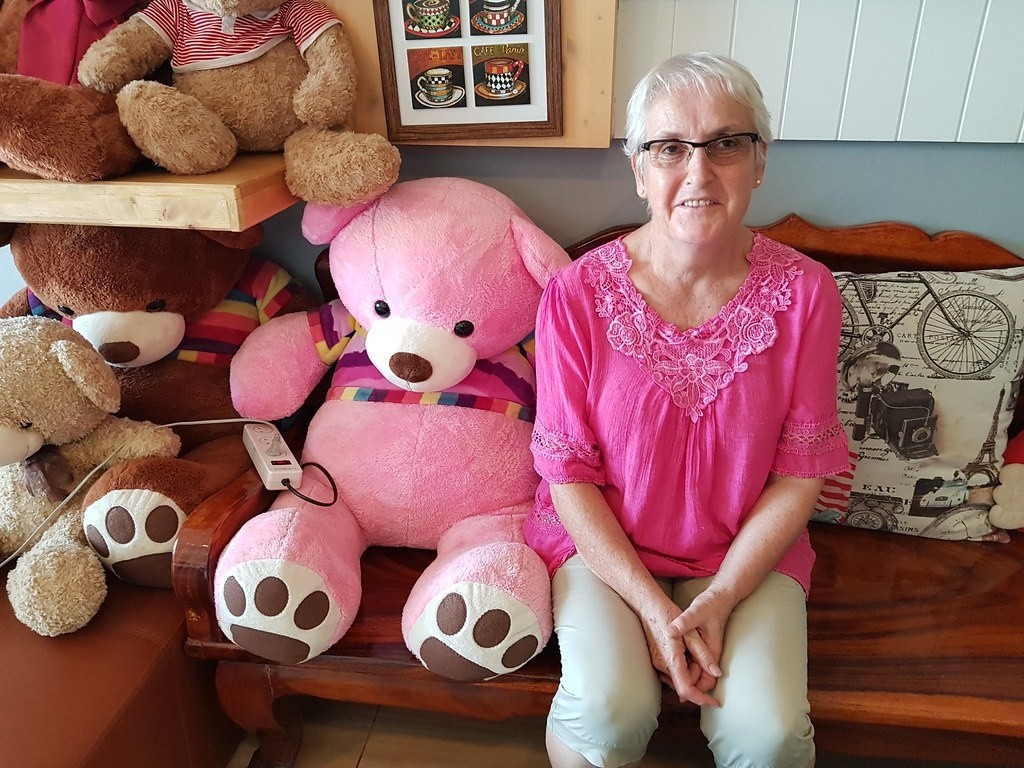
[530,49,850,768]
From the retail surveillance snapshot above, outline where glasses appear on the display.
[638,132,758,170]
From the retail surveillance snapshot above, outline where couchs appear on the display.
[171,211,1024,768]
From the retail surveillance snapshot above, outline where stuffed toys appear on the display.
[77,1,402,208]
[4,224,299,536]
[0,0,140,181]
[1,319,185,639]
[214,176,576,684]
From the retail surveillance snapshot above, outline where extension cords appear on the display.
[242,423,301,492]
